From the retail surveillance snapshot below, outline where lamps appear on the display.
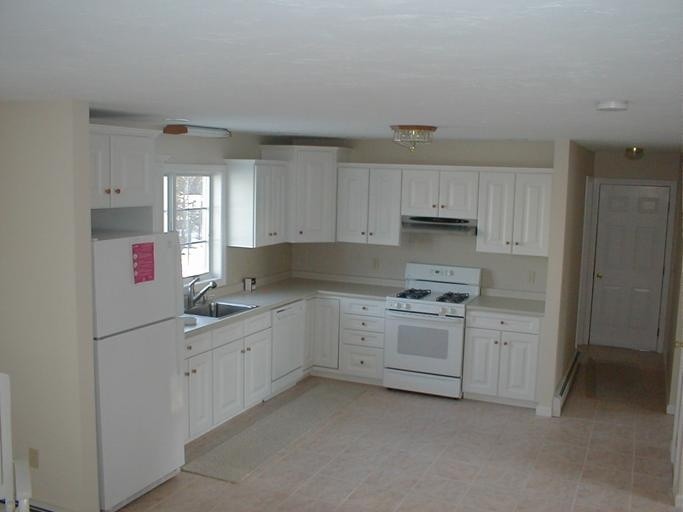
[390,125,437,151]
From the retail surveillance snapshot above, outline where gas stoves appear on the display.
[385,287,480,318]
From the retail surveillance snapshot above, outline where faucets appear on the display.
[187,277,217,308]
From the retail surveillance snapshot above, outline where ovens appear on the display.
[383,310,463,398]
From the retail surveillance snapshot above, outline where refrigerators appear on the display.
[91,228,186,512]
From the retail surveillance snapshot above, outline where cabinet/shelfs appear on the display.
[272,297,309,393]
[476,166,551,257]
[216,312,270,423]
[184,328,215,446]
[340,296,385,387]
[311,294,339,379]
[89,124,159,208]
[402,164,478,222]
[261,145,350,244]
[337,164,402,244]
[225,159,285,249]
[464,308,541,407]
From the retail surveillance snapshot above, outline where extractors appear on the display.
[401,216,476,236]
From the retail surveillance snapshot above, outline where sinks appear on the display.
[184,302,248,318]
